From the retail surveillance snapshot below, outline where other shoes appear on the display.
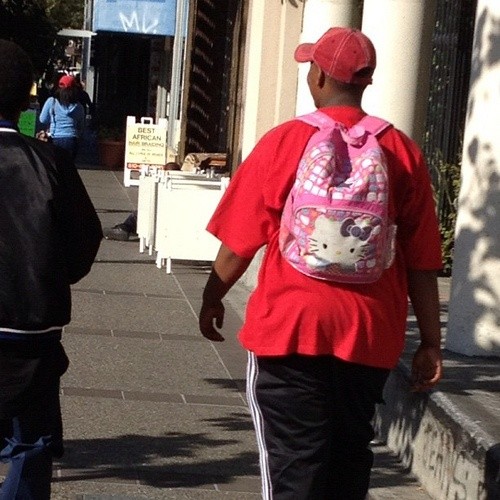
[102,226,129,241]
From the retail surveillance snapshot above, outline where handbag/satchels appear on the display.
[35,130,50,143]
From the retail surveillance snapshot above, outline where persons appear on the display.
[39,76,85,167]
[0,40,102,500]
[199,27,444,500]
[102,162,180,242]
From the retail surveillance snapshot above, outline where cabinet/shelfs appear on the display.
[137,166,231,274]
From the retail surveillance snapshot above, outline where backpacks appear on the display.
[278,108,398,285]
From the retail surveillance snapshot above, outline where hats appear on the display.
[58,75,77,89]
[294,25,378,87]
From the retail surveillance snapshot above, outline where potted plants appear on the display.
[95,127,126,169]
